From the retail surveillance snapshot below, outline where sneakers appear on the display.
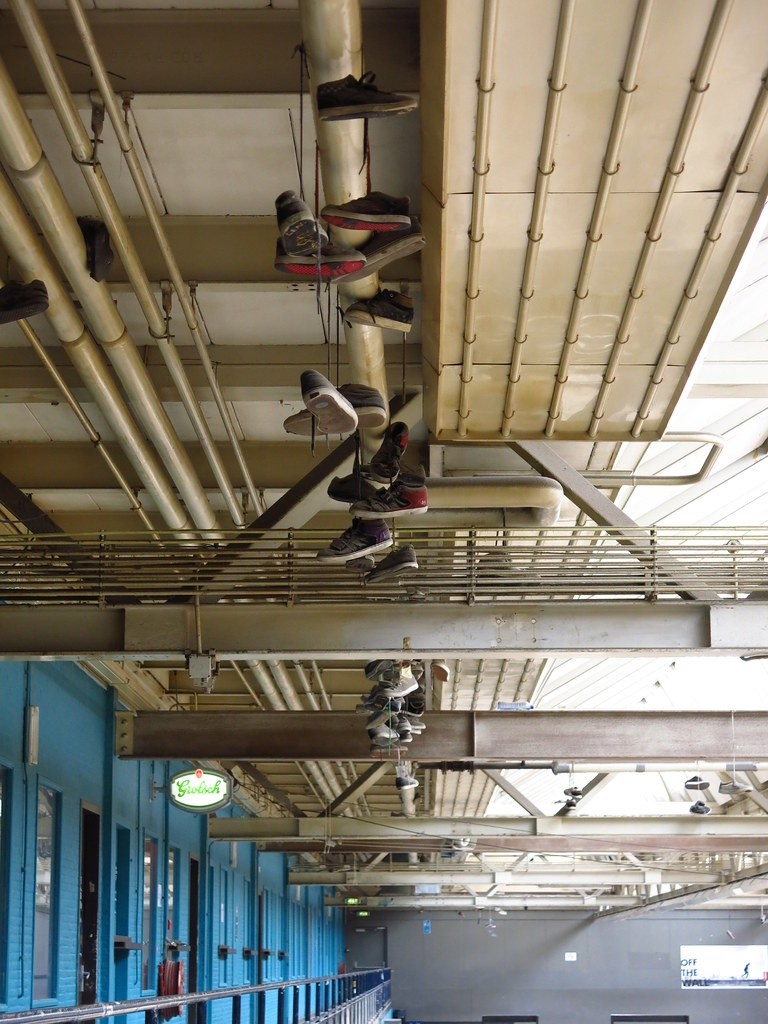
[321,191,411,231]
[346,555,374,572]
[338,215,426,282]
[317,71,417,122]
[718,781,752,794]
[370,421,410,483]
[274,235,367,276]
[316,519,394,563]
[684,776,709,790]
[564,801,577,808]
[349,481,428,520]
[396,776,419,790]
[365,545,419,584]
[689,801,710,814]
[274,190,328,257]
[564,786,583,798]
[282,369,385,435]
[327,472,377,503]
[345,288,414,333]
[355,660,427,758]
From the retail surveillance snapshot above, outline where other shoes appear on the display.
[431,660,449,682]
[413,792,421,804]
